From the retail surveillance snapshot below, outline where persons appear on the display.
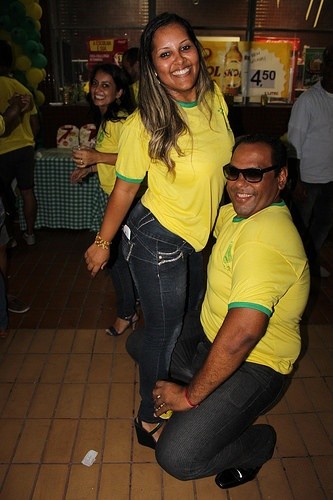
[285,43,333,326]
[0,201,30,339]
[152,130,312,490]
[83,10,238,450]
[0,39,41,247]
[70,64,149,338]
[121,47,142,108]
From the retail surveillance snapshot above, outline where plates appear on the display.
[48,103,63,106]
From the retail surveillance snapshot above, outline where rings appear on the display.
[80,158,83,164]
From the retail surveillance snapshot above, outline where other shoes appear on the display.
[320,266,331,277]
[6,294,31,313]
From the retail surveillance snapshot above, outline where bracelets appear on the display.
[184,384,200,409]
[95,232,112,250]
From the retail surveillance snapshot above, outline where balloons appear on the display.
[0,0,48,105]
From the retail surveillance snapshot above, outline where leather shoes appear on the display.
[217,427,278,488]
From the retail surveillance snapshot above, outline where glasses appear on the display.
[222,163,276,182]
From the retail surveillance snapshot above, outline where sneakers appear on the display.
[8,236,17,248]
[23,229,36,245]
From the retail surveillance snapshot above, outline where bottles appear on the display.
[222,42,243,96]
[63,87,69,104]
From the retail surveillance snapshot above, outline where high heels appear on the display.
[135,417,166,448]
[105,312,138,336]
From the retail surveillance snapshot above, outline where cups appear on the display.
[74,145,90,165]
[261,96,267,107]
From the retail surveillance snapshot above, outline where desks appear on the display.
[13,147,109,231]
